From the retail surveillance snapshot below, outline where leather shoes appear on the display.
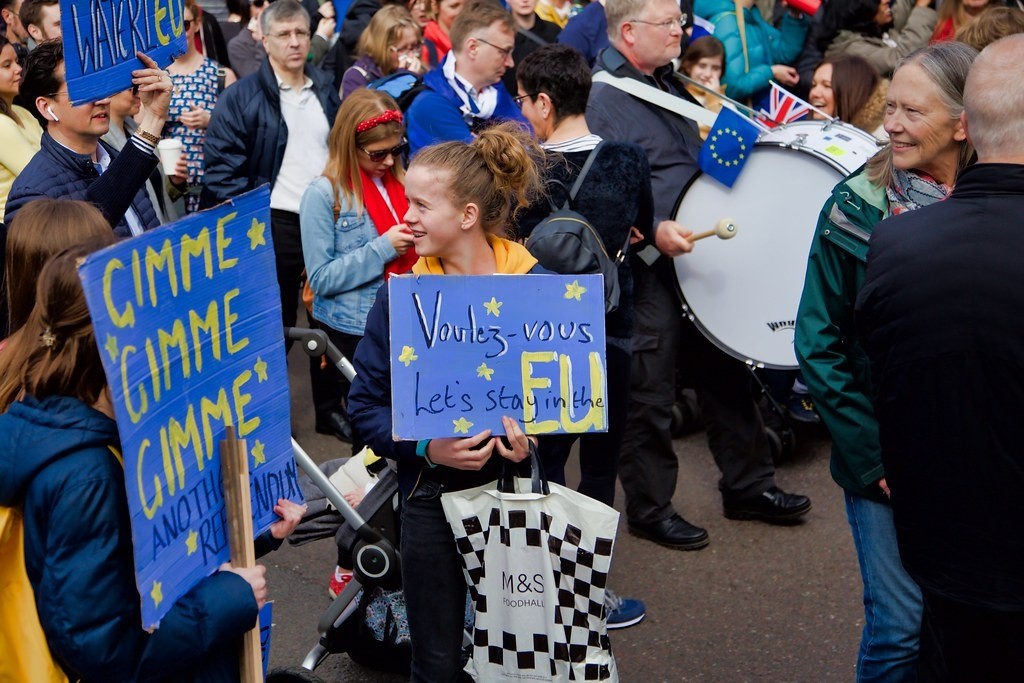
[627,512,710,551]
[722,488,812,523]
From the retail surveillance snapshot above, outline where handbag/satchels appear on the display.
[440,438,619,683]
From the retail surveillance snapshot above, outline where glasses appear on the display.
[356,136,408,162]
[513,92,560,109]
[629,13,688,32]
[476,39,515,58]
[249,0,276,7]
[184,19,195,31]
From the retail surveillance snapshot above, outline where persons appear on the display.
[796,32,1024,683]
[508,44,654,630]
[347,121,560,683]
[0,238,307,683]
[0,0,1024,597]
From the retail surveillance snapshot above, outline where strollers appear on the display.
[262,328,475,683]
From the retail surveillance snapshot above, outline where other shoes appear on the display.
[780,388,820,424]
[328,565,354,600]
[605,588,646,629]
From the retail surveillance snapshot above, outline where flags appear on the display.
[697,103,763,188]
[753,83,813,130]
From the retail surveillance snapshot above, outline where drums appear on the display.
[666,119,887,371]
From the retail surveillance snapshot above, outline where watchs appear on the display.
[416,439,438,469]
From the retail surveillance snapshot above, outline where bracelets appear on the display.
[135,126,160,144]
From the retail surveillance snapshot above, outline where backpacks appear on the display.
[300,172,341,318]
[517,137,644,315]
[0,443,124,683]
[367,69,434,115]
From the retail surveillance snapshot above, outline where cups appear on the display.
[157,139,183,175]
